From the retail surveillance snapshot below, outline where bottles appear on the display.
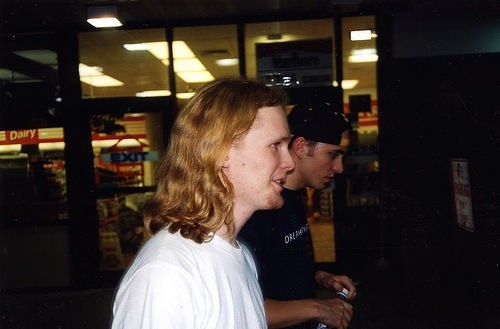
[317,288,348,329]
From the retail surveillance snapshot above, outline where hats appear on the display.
[287,99,351,146]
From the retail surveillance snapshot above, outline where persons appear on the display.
[237,97,358,328]
[106,76,297,328]
